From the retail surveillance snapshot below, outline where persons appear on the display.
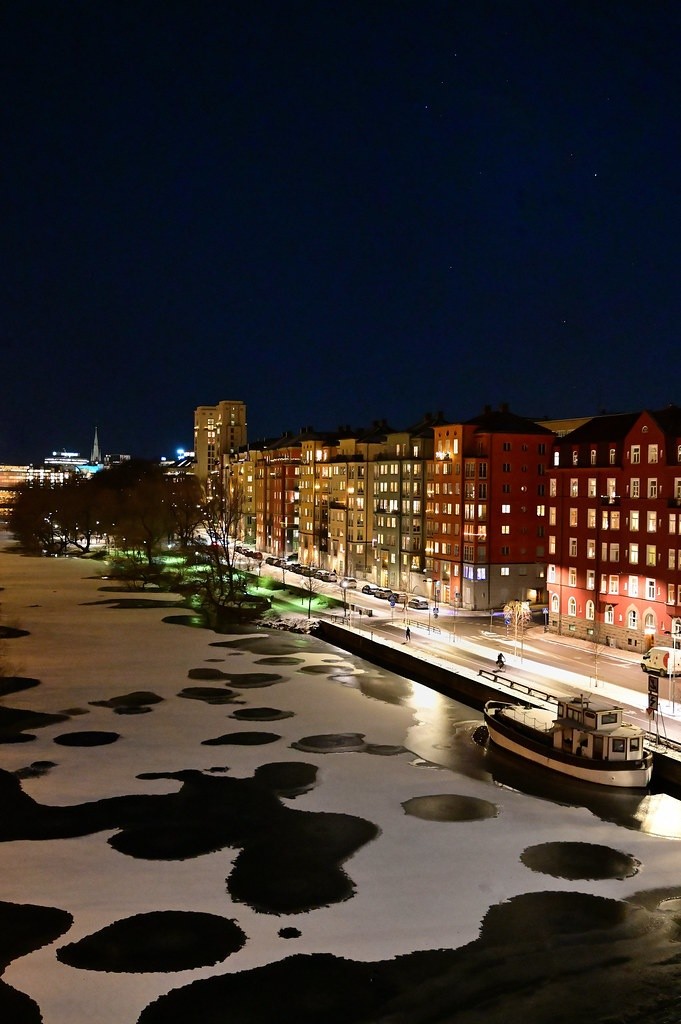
[402,593,408,612]
[405,627,411,641]
[496,652,506,669]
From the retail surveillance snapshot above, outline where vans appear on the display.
[641,647,681,677]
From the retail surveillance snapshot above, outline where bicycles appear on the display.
[495,661,507,672]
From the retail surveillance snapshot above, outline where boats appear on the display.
[483,692,654,788]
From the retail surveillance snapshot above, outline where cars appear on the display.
[340,577,358,588]
[374,589,393,599]
[252,552,262,560]
[361,584,379,595]
[388,592,408,603]
[409,598,429,610]
[234,545,253,556]
[303,567,318,577]
[321,572,337,582]
[265,556,308,574]
[314,570,327,579]
